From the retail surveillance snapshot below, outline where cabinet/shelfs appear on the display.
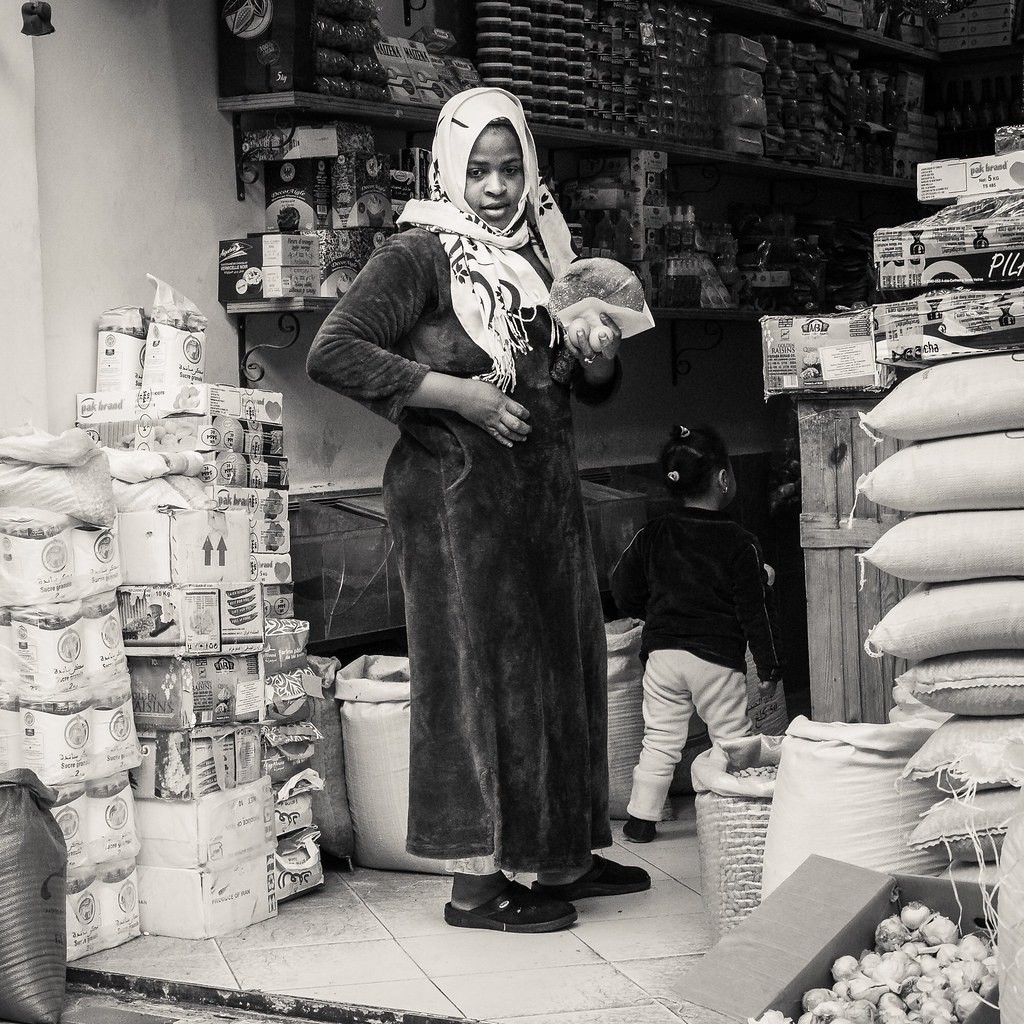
[210,0,941,329]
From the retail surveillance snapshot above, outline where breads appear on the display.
[547,257,643,314]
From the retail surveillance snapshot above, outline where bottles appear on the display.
[666,205,732,252]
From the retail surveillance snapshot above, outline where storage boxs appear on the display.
[80,384,323,938]
[216,231,322,302]
[683,858,1003,1024]
[761,150,1024,401]
[937,0,1016,53]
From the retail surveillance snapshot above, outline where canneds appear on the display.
[582,0,644,139]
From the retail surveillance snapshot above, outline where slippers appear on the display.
[531,854,652,900]
[444,881,578,932]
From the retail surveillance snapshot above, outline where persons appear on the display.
[607,424,786,842]
[305,87,650,934]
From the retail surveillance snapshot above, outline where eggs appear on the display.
[589,325,614,352]
[569,318,590,347]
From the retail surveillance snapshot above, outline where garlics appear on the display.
[750,902,1003,1024]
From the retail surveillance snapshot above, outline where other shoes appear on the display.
[623,816,658,842]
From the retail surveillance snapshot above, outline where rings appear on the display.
[583,354,597,364]
